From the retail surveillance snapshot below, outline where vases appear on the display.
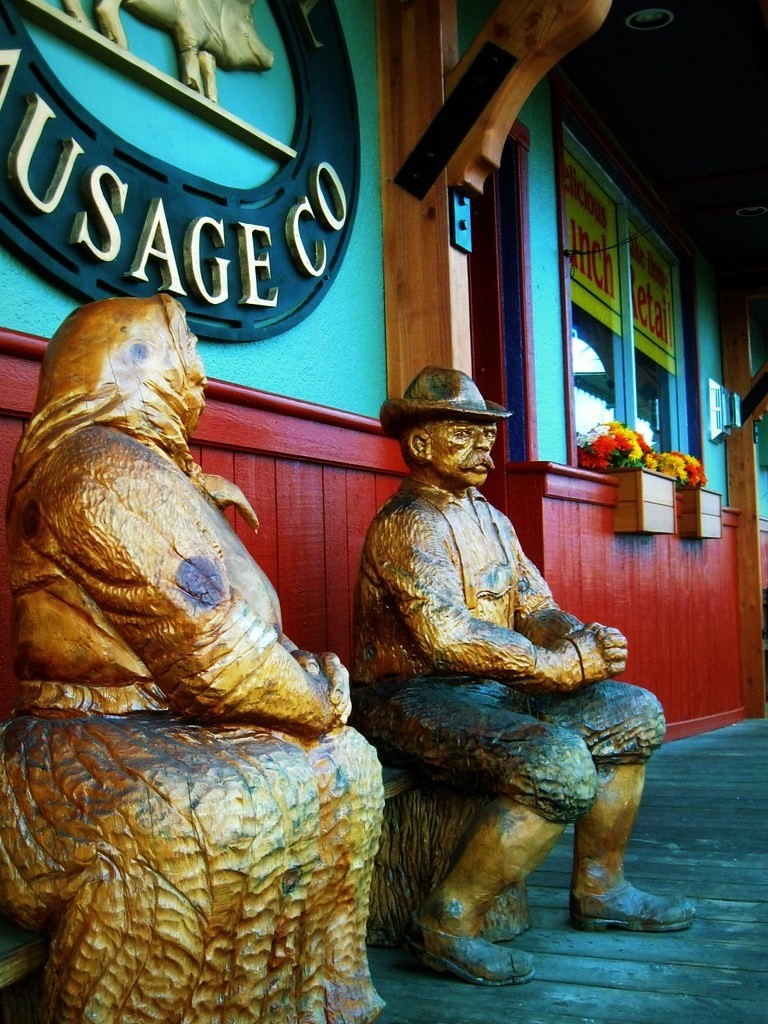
[675,490,723,539]
[599,466,678,535]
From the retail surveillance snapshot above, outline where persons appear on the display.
[0,293,390,1024]
[350,366,695,986]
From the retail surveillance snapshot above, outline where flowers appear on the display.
[577,417,708,487]
